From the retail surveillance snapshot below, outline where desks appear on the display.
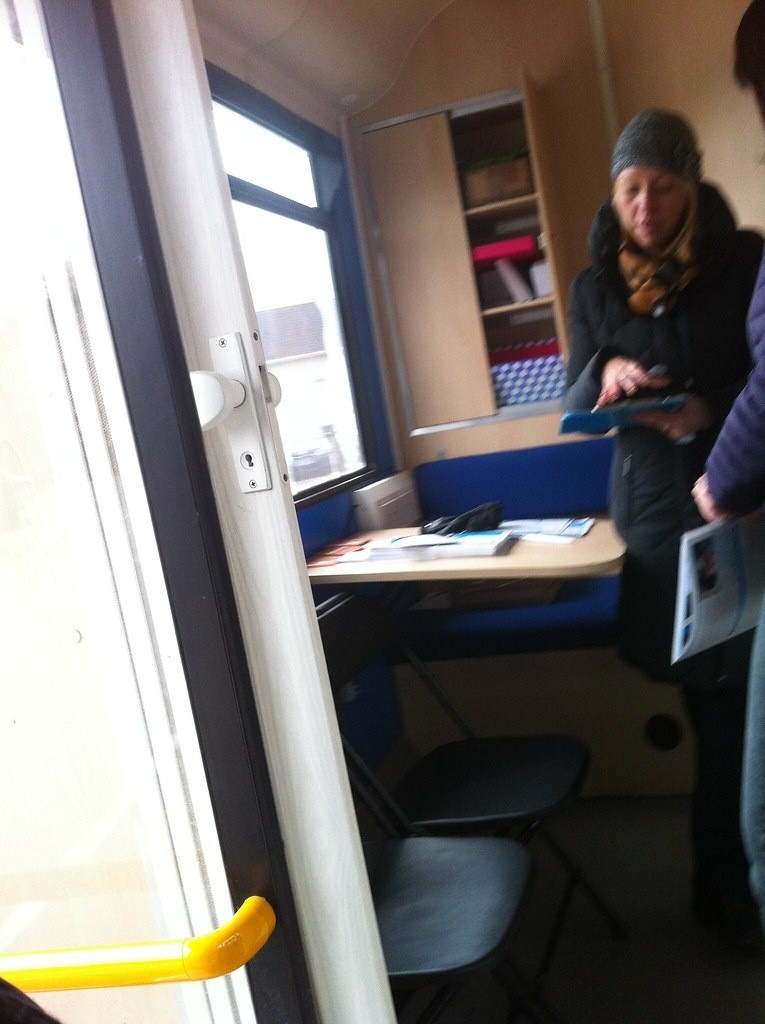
[302,515,628,586]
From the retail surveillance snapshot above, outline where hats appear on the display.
[610,106,702,187]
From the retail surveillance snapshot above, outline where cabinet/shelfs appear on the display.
[345,62,598,429]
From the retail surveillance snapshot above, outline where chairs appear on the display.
[338,731,575,1024]
[313,589,639,941]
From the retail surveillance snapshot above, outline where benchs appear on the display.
[390,435,623,660]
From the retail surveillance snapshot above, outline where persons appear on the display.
[553,105,762,697]
[681,2,765,914]
